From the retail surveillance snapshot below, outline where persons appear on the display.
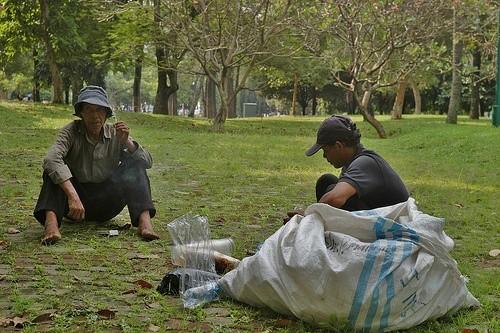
[282,114,410,226]
[17,91,33,101]
[33,84,160,244]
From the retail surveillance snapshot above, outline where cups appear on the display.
[171,239,241,273]
[104,122,117,138]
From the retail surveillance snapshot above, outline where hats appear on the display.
[305,114,362,156]
[72,85,114,119]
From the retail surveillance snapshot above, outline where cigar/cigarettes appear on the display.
[114,119,117,136]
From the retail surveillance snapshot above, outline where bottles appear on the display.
[182,282,220,309]
